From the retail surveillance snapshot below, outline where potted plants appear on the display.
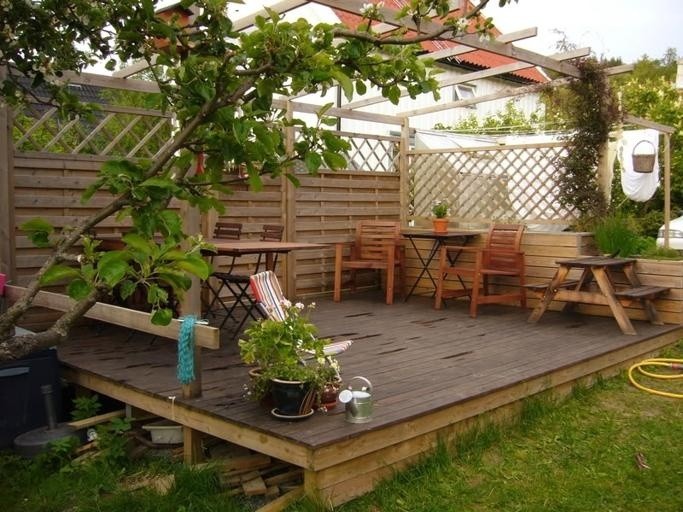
[430,202,457,228]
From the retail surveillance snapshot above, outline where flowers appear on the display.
[238,298,343,415]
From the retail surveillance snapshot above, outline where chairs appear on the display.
[435,223,528,317]
[248,270,355,386]
[125,221,285,346]
[334,219,406,306]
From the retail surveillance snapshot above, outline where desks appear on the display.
[153,235,332,356]
[527,256,664,335]
[399,230,482,308]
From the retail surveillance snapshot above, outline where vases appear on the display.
[248,363,343,423]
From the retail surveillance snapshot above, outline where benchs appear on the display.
[521,277,672,335]
